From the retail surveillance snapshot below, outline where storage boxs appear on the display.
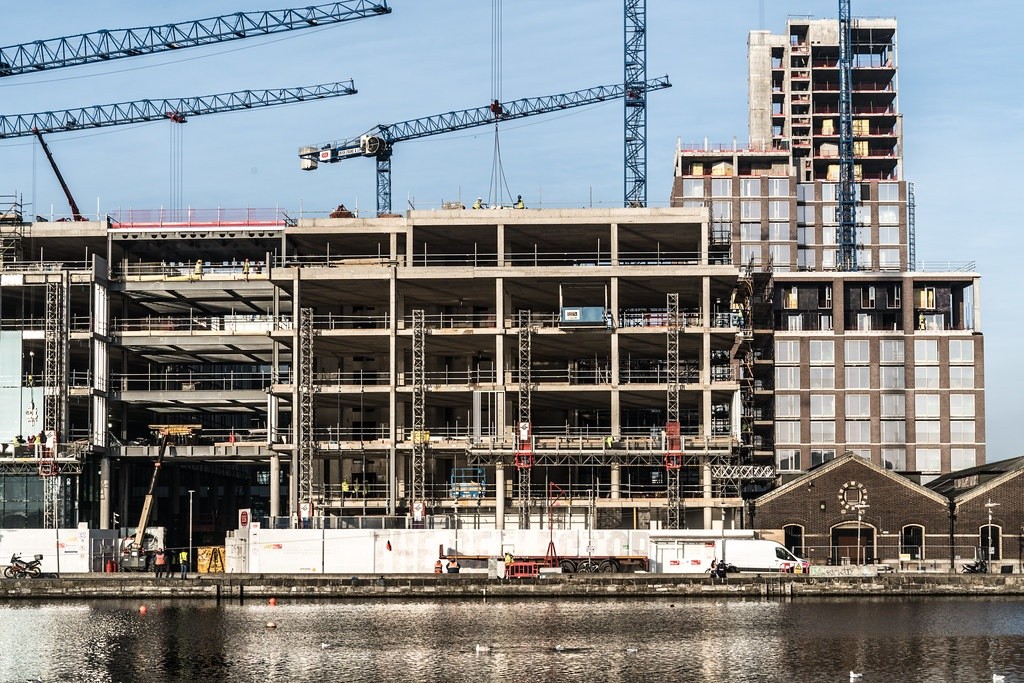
[34,554,44,560]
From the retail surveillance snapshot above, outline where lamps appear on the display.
[820,499,826,512]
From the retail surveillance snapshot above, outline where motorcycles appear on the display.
[960,560,988,574]
[3,552,43,579]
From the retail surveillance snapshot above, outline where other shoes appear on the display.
[185,578,188,580]
[181,577,183,580]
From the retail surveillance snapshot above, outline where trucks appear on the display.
[713,538,810,575]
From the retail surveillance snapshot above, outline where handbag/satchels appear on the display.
[182,560,188,565]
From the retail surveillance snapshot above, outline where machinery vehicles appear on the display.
[115,425,169,573]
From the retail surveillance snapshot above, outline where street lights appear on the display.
[854,503,871,566]
[188,490,196,573]
[984,497,1001,574]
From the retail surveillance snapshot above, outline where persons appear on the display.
[342,479,350,498]
[242,258,250,274]
[435,560,443,574]
[446,558,460,573]
[513,195,524,209]
[150,548,167,578]
[166,550,178,578]
[716,560,727,578]
[18,430,47,457]
[351,478,370,498]
[472,198,483,209]
[195,259,203,275]
[505,550,514,562]
[710,560,716,573]
[178,548,189,580]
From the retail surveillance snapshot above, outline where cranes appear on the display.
[0,1,393,81]
[0,79,357,142]
[297,72,673,219]
